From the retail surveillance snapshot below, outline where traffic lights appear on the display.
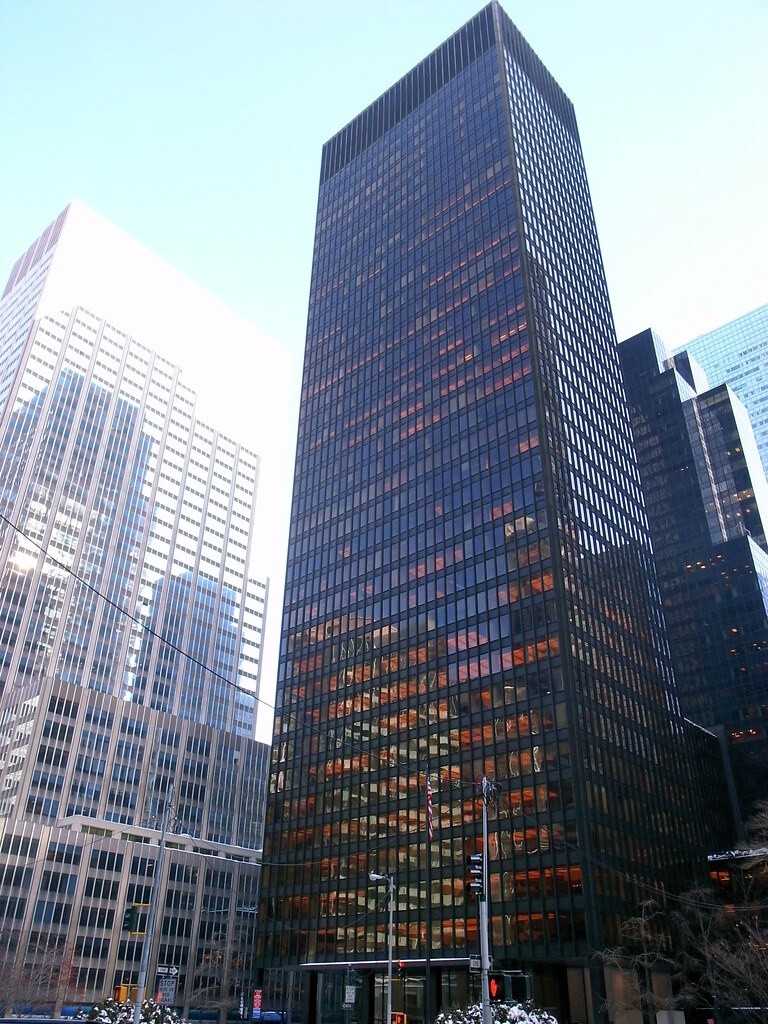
[393,1014,406,1024]
[122,906,138,932]
[488,973,506,1004]
[466,853,483,894]
[114,986,127,1001]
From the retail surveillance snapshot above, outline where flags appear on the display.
[426,776,433,841]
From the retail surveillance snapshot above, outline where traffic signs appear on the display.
[341,1002,354,1010]
[156,964,180,977]
[470,954,493,972]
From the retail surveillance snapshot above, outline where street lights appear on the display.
[370,874,393,1024]
